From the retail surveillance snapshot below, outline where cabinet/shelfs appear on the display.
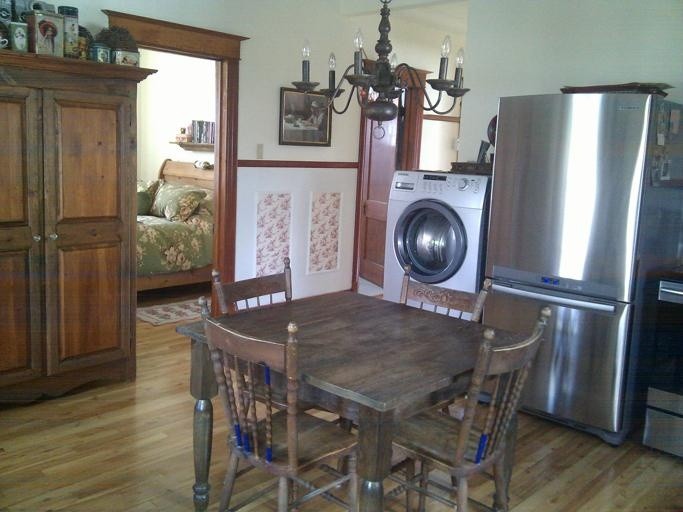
[0,48,157,405]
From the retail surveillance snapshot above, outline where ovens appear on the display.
[642,283,682,461]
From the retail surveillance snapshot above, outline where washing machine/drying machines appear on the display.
[381,169,492,325]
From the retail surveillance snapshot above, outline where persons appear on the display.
[297,100,326,133]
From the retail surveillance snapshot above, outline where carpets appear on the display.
[137,296,212,326]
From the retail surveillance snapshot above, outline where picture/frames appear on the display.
[280,87,331,146]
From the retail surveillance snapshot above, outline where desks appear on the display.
[174,289,547,512]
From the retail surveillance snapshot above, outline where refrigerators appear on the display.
[470,91,683,448]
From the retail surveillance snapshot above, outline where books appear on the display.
[175,119,215,144]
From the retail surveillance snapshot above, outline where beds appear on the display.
[137,159,214,292]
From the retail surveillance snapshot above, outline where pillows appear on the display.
[137,178,206,223]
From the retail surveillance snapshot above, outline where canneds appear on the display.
[88,43,111,64]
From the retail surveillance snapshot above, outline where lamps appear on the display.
[292,0,471,141]
